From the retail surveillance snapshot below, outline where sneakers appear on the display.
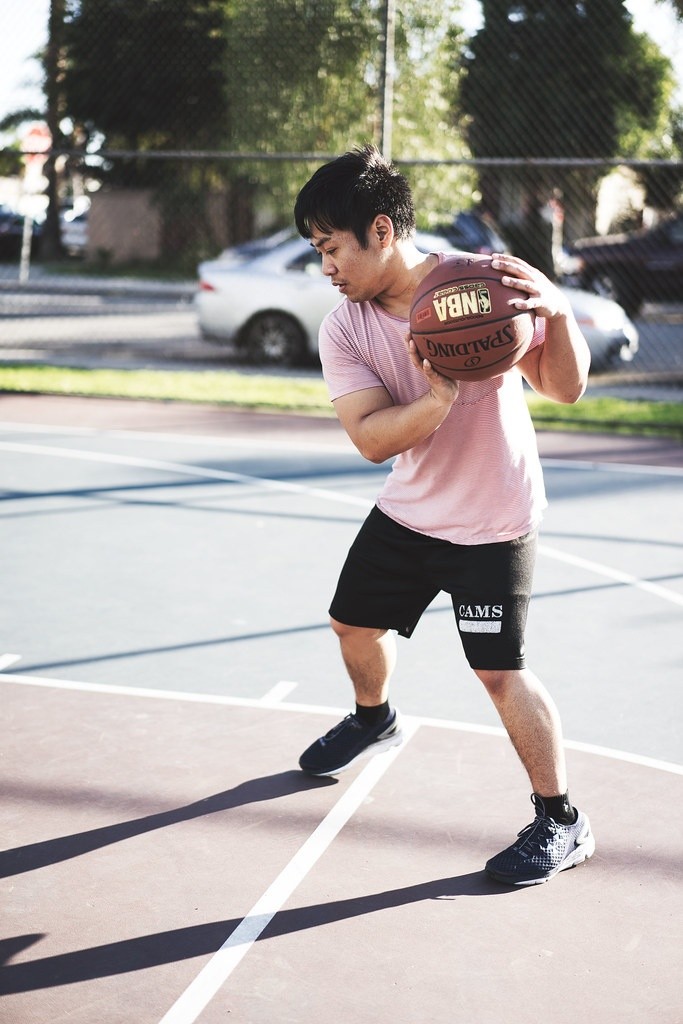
[299,706,404,776]
[486,793,595,885]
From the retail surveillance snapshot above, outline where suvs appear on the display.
[572,215,683,311]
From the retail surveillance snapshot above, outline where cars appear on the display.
[0,197,90,260]
[197,212,640,375]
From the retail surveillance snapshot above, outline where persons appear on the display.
[505,193,555,282]
[293,147,596,886]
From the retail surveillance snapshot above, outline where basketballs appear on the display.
[410,256,535,382]
